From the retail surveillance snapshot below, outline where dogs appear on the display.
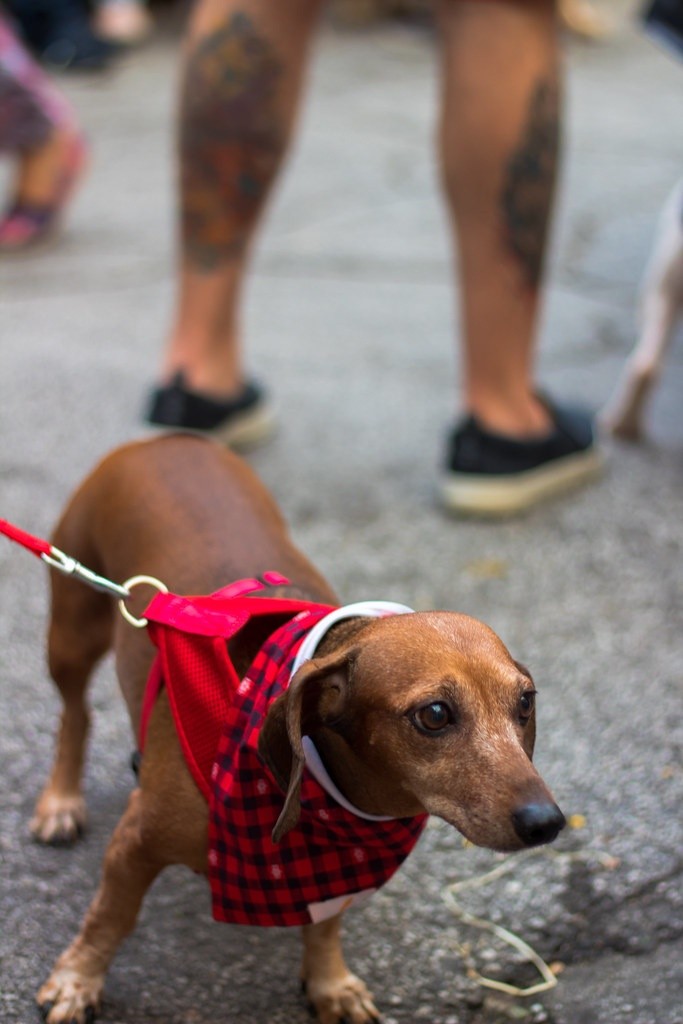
[25,430,566,1024]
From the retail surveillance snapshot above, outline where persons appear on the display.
[135,0,616,520]
[604,174,683,450]
[0,0,147,254]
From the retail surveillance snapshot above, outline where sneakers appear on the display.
[437,387,600,516]
[136,367,280,448]
